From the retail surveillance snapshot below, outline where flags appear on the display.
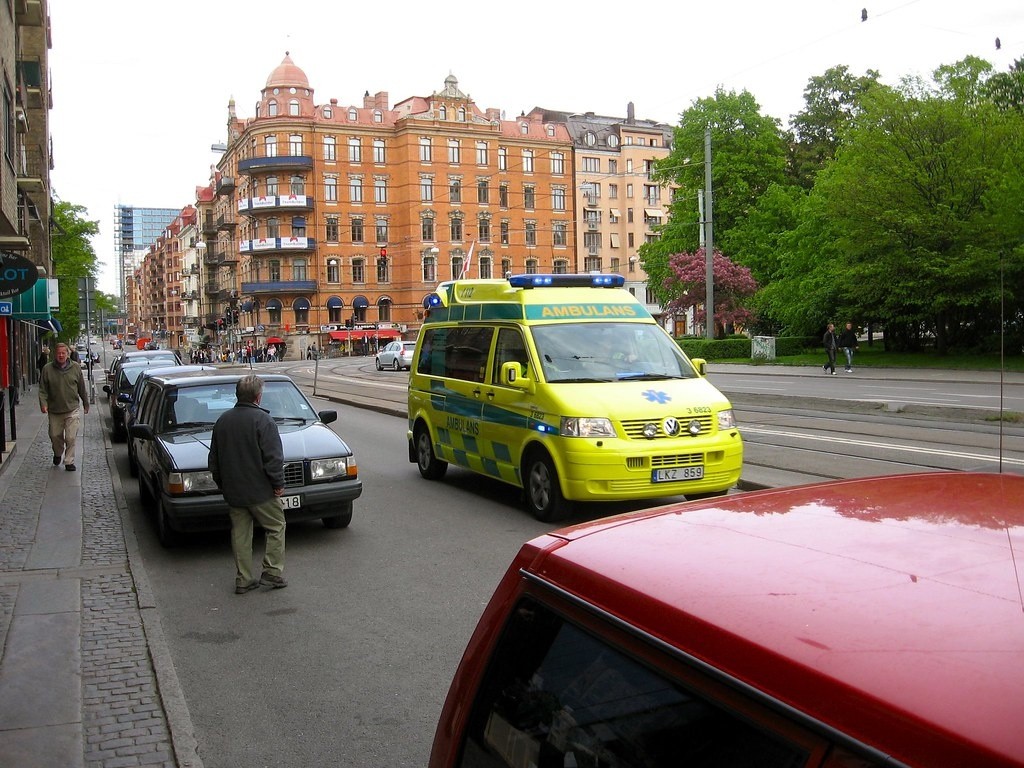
[463,241,474,272]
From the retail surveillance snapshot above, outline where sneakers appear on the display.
[845,369,852,373]
[53,455,62,466]
[65,463,76,471]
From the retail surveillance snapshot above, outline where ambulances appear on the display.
[407,273,744,526]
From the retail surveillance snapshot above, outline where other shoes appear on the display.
[822,365,828,374]
[235,578,262,594]
[258,572,288,588]
[363,355,365,358]
[831,372,837,375]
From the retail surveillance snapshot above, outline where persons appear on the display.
[838,321,859,372]
[362,332,369,357]
[369,334,377,356]
[594,336,637,363]
[37,343,94,471]
[175,341,287,364]
[208,375,288,594]
[307,342,317,360]
[822,323,840,374]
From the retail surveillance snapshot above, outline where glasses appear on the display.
[43,346,46,348]
[45,353,49,356]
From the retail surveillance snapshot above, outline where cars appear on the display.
[426,471,1024,768]
[125,367,363,549]
[117,365,221,479]
[92,351,101,363]
[101,332,157,350]
[75,351,92,369]
[102,360,181,443]
[104,352,124,398]
[109,350,185,389]
[75,335,97,351]
[375,340,418,372]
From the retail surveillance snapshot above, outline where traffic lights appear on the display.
[232,310,238,324]
[351,313,358,328]
[226,311,232,324]
[380,248,386,268]
[221,316,227,329]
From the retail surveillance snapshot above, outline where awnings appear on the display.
[12,278,51,321]
[38,316,63,339]
